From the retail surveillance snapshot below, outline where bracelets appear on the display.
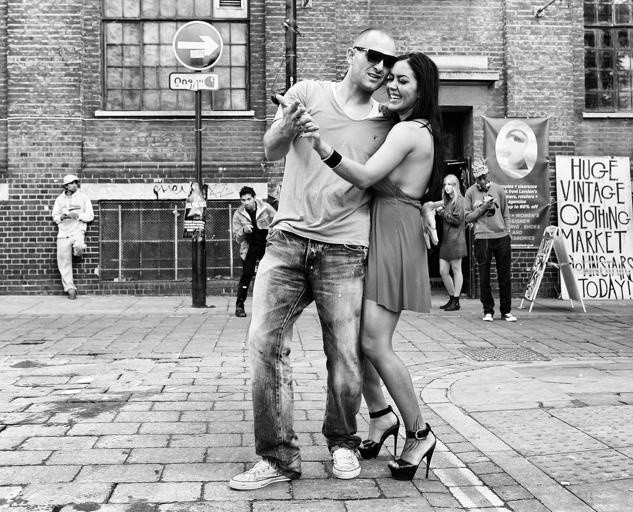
[320,148,343,171]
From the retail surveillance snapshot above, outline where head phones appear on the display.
[475,180,490,192]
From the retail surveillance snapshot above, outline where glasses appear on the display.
[353,46,397,68]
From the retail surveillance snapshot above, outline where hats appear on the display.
[62,175,80,185]
[473,165,489,178]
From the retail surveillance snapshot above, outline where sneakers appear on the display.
[332,448,362,481]
[501,311,516,322]
[483,313,493,322]
[68,293,77,299]
[229,458,291,490]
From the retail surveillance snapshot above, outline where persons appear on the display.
[52,174,95,299]
[231,186,276,318]
[436,175,467,311]
[464,161,518,322]
[288,51,437,483]
[229,25,445,492]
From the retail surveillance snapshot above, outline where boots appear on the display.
[235,283,250,317]
[440,295,460,311]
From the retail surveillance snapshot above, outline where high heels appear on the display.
[358,405,400,460]
[387,423,436,480]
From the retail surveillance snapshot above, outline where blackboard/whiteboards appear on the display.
[523,226,557,302]
